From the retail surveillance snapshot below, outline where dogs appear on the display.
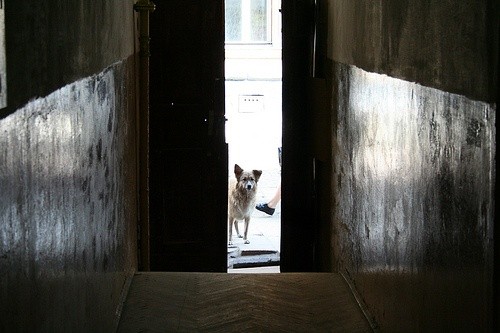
[228,163,263,245]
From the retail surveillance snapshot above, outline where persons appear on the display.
[256,184,281,215]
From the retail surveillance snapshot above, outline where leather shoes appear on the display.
[254,202,275,215]
